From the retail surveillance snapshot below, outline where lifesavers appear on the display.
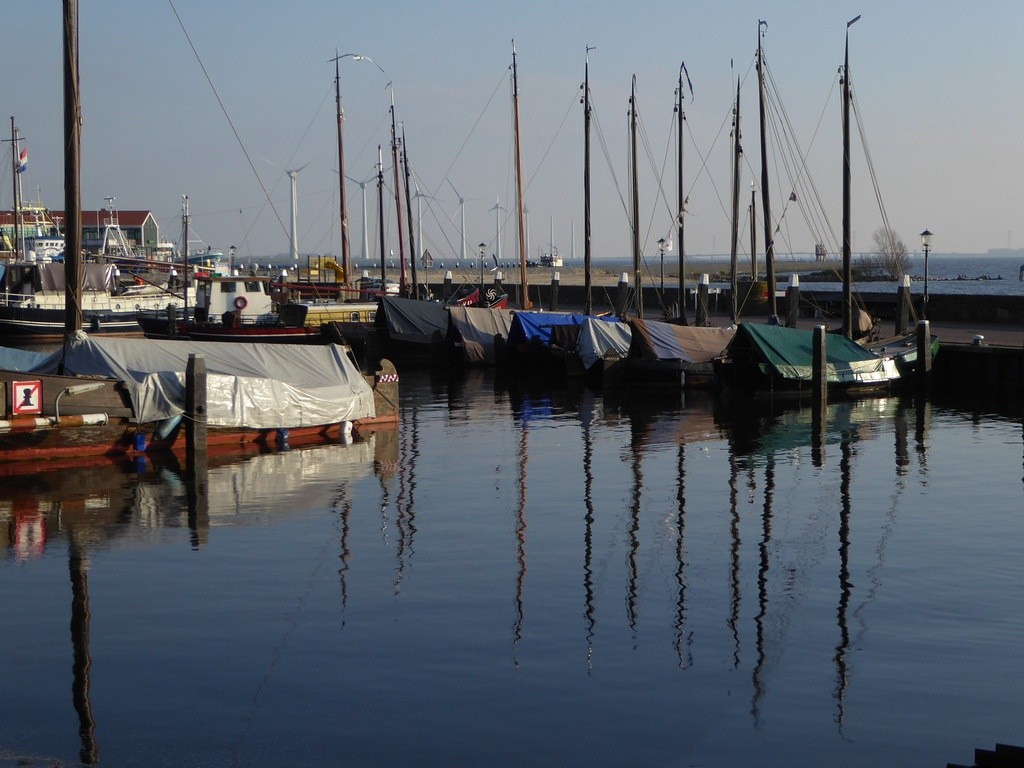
[134,278,143,285]
[234,296,248,310]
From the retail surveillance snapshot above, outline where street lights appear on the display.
[657,237,667,307]
[229,245,237,276]
[919,229,936,320]
[477,242,488,304]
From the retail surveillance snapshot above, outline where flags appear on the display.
[16,148,27,173]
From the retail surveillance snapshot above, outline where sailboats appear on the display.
[0,12,943,400]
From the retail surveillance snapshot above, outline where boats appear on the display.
[0,333,383,478]
